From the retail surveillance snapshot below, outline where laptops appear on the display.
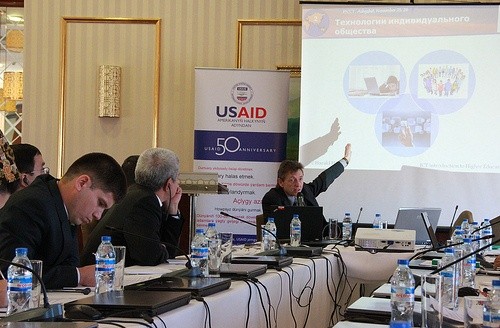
[229,255,294,268]
[342,252,445,327]
[209,262,267,281]
[393,208,441,242]
[421,210,450,252]
[273,204,324,244]
[123,276,232,297]
[275,247,323,256]
[63,289,191,317]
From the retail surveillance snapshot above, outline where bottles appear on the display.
[264,218,276,249]
[373,214,382,228]
[483,279,500,328]
[95,236,116,294]
[204,223,219,271]
[290,215,301,247]
[342,213,352,240]
[7,248,32,315]
[191,228,209,277]
[439,217,493,311]
[390,258,413,328]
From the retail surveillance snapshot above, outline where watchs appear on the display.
[171,209,180,216]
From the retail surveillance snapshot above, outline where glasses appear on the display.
[31,167,50,175]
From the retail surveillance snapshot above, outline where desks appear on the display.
[0,240,500,328]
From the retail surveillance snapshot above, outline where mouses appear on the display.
[65,304,102,319]
[458,287,479,297]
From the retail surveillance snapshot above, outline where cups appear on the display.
[219,232,232,264]
[464,296,491,328]
[113,246,125,290]
[30,260,42,308]
[329,219,338,239]
[261,225,272,250]
[421,274,443,328]
[378,221,387,229]
[207,239,220,277]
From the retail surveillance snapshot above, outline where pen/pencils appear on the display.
[0,270,6,281]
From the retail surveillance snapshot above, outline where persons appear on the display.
[399,128,415,146]
[421,65,465,96]
[262,144,352,238]
[122,155,140,187]
[10,144,50,192]
[0,152,127,308]
[379,76,399,92]
[83,148,182,267]
[0,129,20,211]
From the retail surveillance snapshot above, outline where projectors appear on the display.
[355,227,417,252]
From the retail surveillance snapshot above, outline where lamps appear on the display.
[6,14,25,22]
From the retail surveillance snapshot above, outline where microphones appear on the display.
[408,234,496,262]
[448,205,459,240]
[222,211,286,256]
[102,224,201,275]
[346,207,364,246]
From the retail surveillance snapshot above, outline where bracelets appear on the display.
[343,157,349,164]
